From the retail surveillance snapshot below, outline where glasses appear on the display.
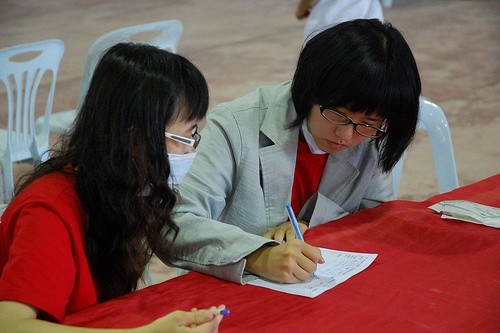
[164,123,201,149]
[319,104,389,139]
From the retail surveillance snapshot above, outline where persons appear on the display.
[296,0,392,46]
[148,17,422,284]
[1,42,225,332]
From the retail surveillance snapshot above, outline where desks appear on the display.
[60,174,500,333]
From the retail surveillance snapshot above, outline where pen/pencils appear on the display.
[286,204,315,276]
[197,309,230,315]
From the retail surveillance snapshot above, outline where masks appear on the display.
[168,153,198,191]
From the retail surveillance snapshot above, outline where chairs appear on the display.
[0,20,460,288]
